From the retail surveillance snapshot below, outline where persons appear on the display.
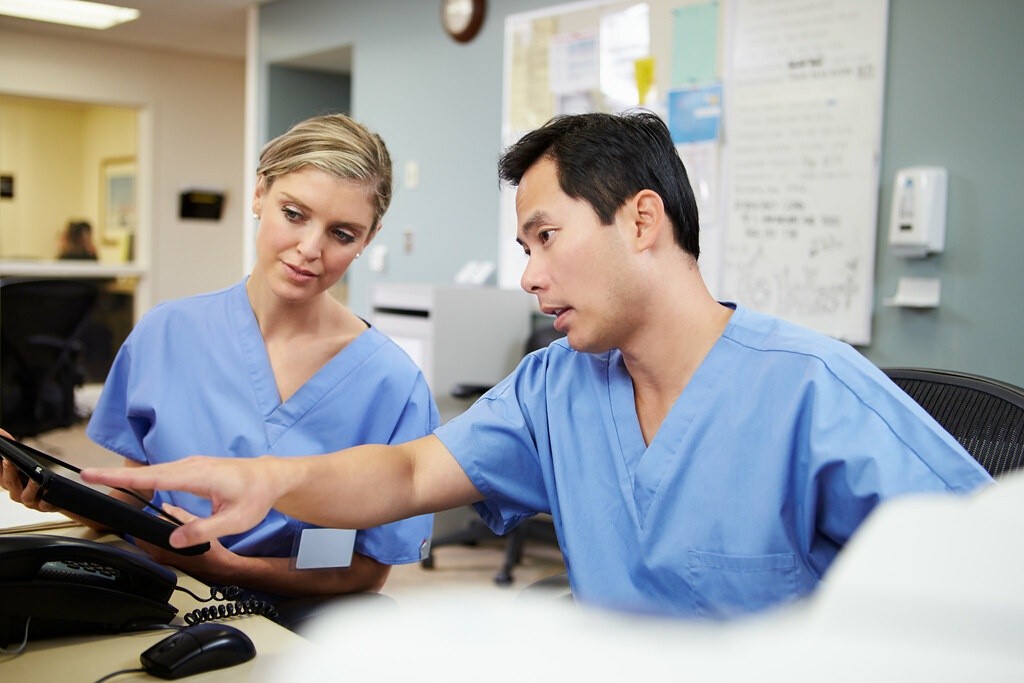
[0,113,444,597]
[79,112,999,623]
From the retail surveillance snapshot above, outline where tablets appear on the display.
[0,434,212,556]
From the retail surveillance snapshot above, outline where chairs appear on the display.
[422,311,554,584]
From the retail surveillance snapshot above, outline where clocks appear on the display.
[441,0,485,42]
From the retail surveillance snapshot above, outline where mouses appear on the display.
[139,622,257,679]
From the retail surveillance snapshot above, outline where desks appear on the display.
[0,487,310,683]
